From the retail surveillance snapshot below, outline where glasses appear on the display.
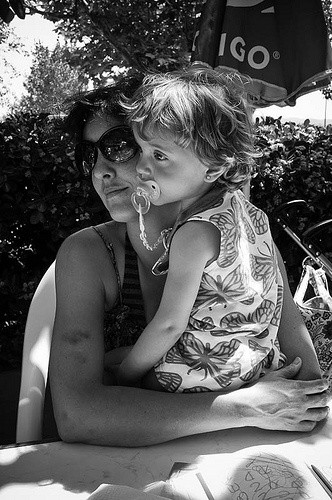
[75,125,138,177]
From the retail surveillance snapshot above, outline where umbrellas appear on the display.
[192,0,332,205]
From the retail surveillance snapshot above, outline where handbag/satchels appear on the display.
[293,265,332,398]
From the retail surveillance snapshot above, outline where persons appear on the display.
[50,66,330,448]
